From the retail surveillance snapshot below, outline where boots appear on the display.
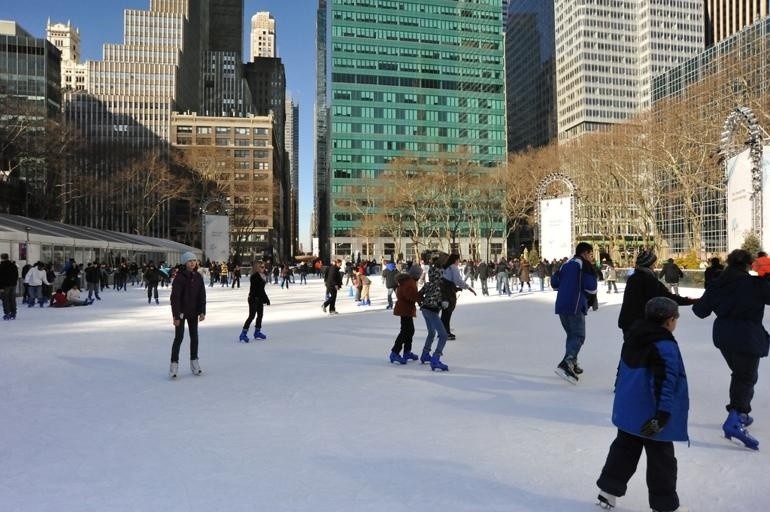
[357,299,370,306]
[191,359,201,376]
[254,330,266,339]
[240,331,249,343]
[389,349,447,370]
[170,363,179,377]
[557,355,583,381]
[723,409,759,449]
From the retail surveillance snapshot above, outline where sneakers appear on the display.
[323,303,338,314]
[597,489,616,507]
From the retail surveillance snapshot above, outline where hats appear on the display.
[646,297,679,319]
[181,252,198,265]
[636,252,656,267]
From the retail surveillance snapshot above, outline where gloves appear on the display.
[640,410,670,438]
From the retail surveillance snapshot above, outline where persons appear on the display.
[751,251,770,278]
[660,257,683,297]
[595,297,690,512]
[705,258,724,293]
[618,251,699,342]
[692,249,770,448]
